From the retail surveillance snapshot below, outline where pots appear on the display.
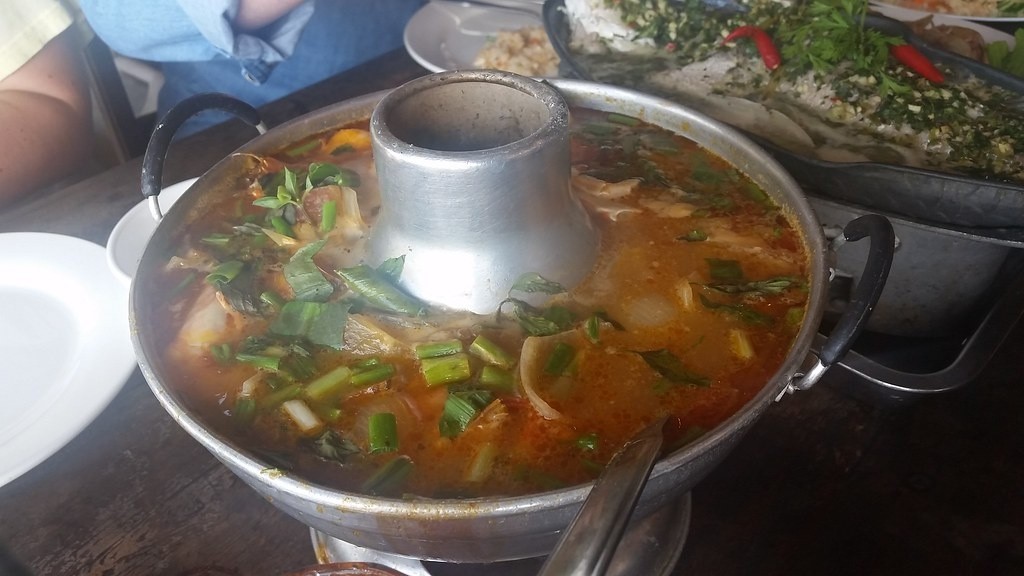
[126,67,897,576]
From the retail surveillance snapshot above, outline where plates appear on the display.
[531,0,1024,253]
[397,4,557,79]
[1,225,135,493]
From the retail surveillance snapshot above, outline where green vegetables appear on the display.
[714,0,912,96]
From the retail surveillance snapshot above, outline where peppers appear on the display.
[721,24,782,72]
[886,35,944,85]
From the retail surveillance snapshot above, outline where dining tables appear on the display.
[0,44,1024,576]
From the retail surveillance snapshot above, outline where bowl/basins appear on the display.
[103,178,196,283]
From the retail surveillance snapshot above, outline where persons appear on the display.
[76,1,428,145]
